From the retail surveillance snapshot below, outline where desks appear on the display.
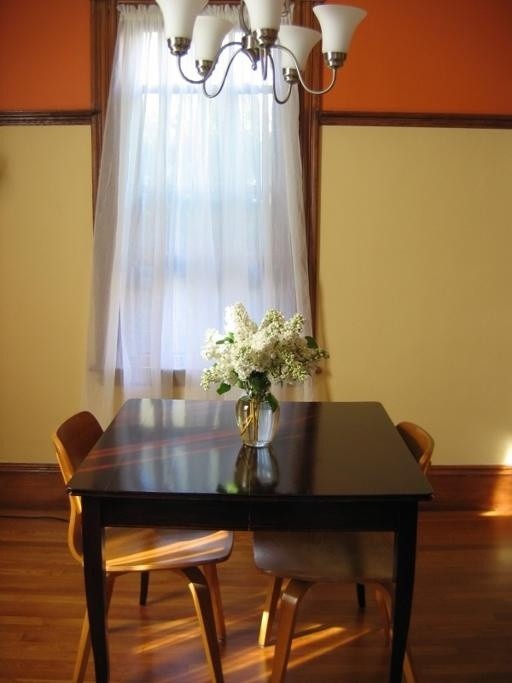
[66,399,434,683]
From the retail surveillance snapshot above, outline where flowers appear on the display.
[200,302,330,442]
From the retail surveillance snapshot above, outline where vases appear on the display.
[235,389,281,449]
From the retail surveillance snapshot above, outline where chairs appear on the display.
[51,410,236,682]
[250,421,434,682]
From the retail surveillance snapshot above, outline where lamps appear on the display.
[155,0,367,103]
